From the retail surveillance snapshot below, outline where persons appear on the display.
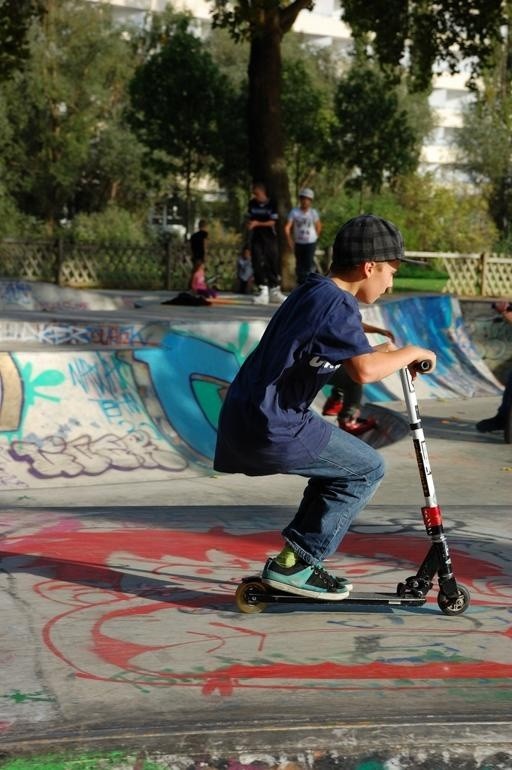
[189,219,210,290]
[283,188,321,287]
[190,260,210,298]
[237,247,254,293]
[246,185,288,305]
[214,214,436,600]
[476,300,511,443]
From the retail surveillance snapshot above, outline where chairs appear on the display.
[322,397,344,416]
[261,557,350,601]
[339,408,376,435]
[315,563,354,591]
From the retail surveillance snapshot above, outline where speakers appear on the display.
[269,284,288,304]
[251,285,270,305]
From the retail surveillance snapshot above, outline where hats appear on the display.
[247,200,280,221]
[154,214,188,237]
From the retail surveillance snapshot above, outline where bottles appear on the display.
[299,187,315,201]
[332,214,427,265]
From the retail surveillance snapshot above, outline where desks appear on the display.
[54,93,76,236]
